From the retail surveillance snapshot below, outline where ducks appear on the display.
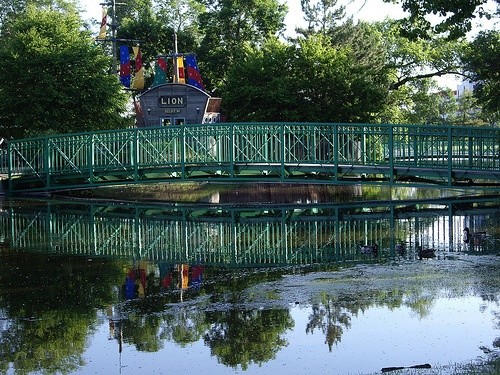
[395,241,407,251]
[418,246,436,257]
[463,227,487,236]
[359,241,379,254]
